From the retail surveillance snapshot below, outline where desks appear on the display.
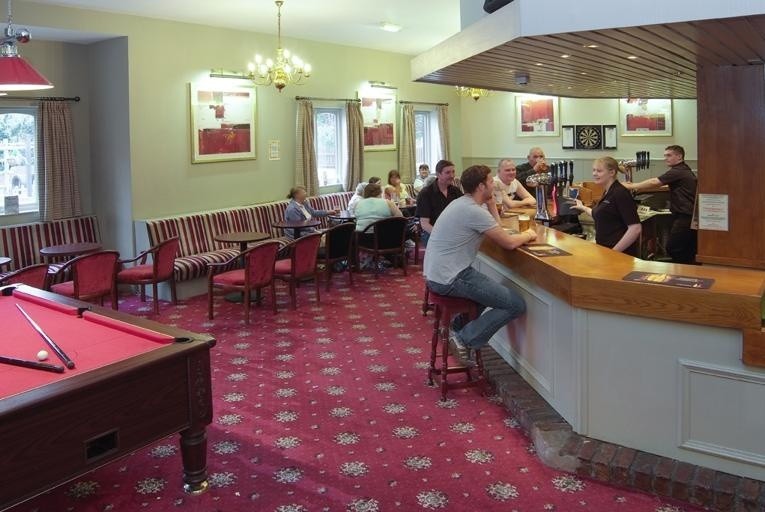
[1,283,216,511]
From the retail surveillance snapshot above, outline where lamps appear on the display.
[246,2,314,95]
[1,0,55,93]
[454,86,496,102]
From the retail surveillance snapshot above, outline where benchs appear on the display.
[2,215,102,279]
[130,201,292,303]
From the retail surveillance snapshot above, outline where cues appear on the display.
[15,303,75,369]
[0,356,64,374]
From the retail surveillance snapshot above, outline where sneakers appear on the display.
[403,238,416,248]
[448,336,479,368]
[440,326,457,343]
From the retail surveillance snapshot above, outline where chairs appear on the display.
[356,217,409,278]
[205,240,287,324]
[118,236,178,315]
[415,211,426,265]
[0,257,51,291]
[275,233,322,310]
[318,222,356,289]
[52,250,118,312]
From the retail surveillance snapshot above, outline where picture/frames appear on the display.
[620,97,674,137]
[184,73,259,164]
[514,91,560,136]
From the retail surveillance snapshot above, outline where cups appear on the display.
[333,205,340,218]
[496,202,506,217]
[518,216,530,233]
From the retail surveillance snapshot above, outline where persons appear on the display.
[283,184,337,249]
[516,147,550,199]
[347,182,367,216]
[353,182,405,250]
[481,159,537,210]
[619,144,698,247]
[414,163,430,195]
[384,169,412,206]
[368,175,382,184]
[422,163,537,368]
[665,231,709,267]
[570,156,643,258]
[415,160,463,246]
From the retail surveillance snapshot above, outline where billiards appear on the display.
[37,350,48,360]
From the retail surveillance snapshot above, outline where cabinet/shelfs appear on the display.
[356,85,400,151]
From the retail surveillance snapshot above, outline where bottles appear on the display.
[391,192,416,208]
[550,182,559,217]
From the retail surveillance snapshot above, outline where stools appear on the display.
[422,283,492,402]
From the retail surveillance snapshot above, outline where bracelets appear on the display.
[524,232,534,240]
